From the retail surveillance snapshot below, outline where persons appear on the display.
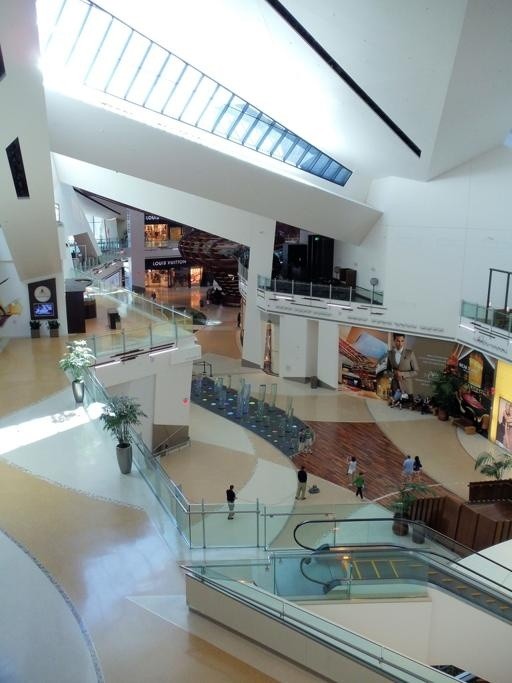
[347,456,357,486]
[303,426,313,454]
[226,485,238,520]
[237,312,241,327]
[352,471,365,499]
[387,333,419,394]
[412,456,423,482]
[298,429,305,453]
[390,388,431,415]
[295,466,307,501]
[496,403,512,451]
[401,456,414,485]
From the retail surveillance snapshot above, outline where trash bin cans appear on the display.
[412,520,425,544]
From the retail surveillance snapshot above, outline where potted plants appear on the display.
[29,320,60,338]
[58,339,98,404]
[390,482,438,536]
[98,393,151,474]
